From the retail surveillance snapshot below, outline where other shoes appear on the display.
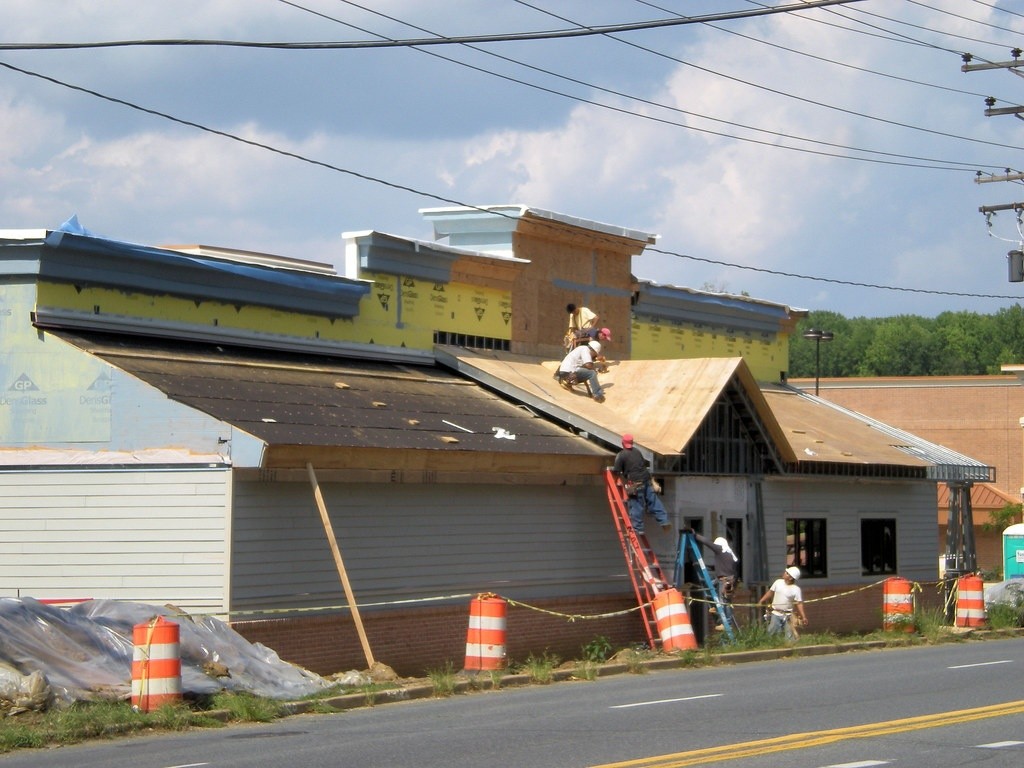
[561,380,576,393]
[662,525,671,532]
[591,394,603,403]
[709,607,717,613]
[715,624,732,631]
[634,529,645,535]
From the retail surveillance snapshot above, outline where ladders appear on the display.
[672,526,741,644]
[602,466,672,654]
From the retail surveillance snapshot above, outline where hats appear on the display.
[622,434,633,448]
[601,328,611,342]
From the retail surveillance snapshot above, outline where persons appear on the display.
[570,324,611,353]
[694,531,738,631]
[759,566,808,645]
[557,339,605,403]
[564,302,596,356]
[613,434,672,536]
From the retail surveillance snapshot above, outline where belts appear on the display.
[773,609,791,613]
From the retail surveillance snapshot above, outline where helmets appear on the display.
[588,341,601,355]
[786,566,801,583]
